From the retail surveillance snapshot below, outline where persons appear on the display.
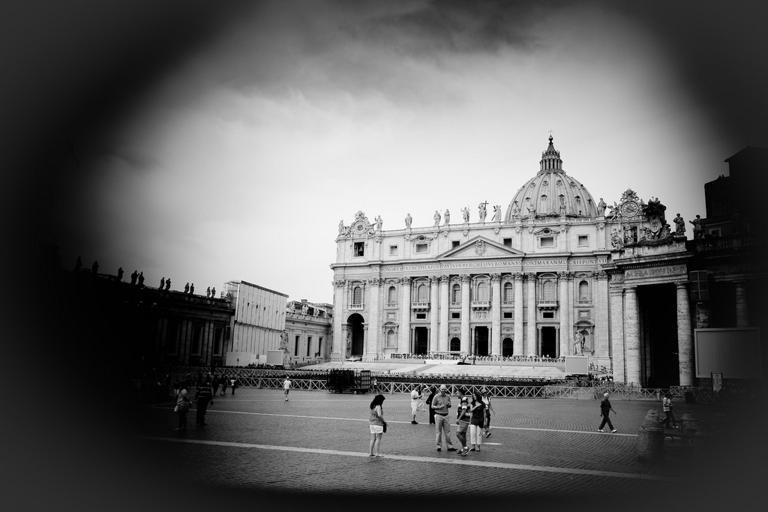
[491,204,500,222]
[479,388,496,439]
[572,331,584,354]
[671,213,687,235]
[429,384,458,452]
[459,206,470,222]
[374,215,383,229]
[595,392,618,434]
[404,212,413,228]
[469,391,486,453]
[443,208,451,224]
[596,197,607,217]
[526,203,536,220]
[558,200,566,218]
[662,391,672,429]
[366,394,387,459]
[478,202,488,221]
[426,388,437,425]
[75,255,217,298]
[154,368,239,438]
[688,213,704,240]
[664,392,678,427]
[433,210,442,227]
[409,384,423,425]
[282,376,293,402]
[454,388,472,457]
[387,350,565,364]
